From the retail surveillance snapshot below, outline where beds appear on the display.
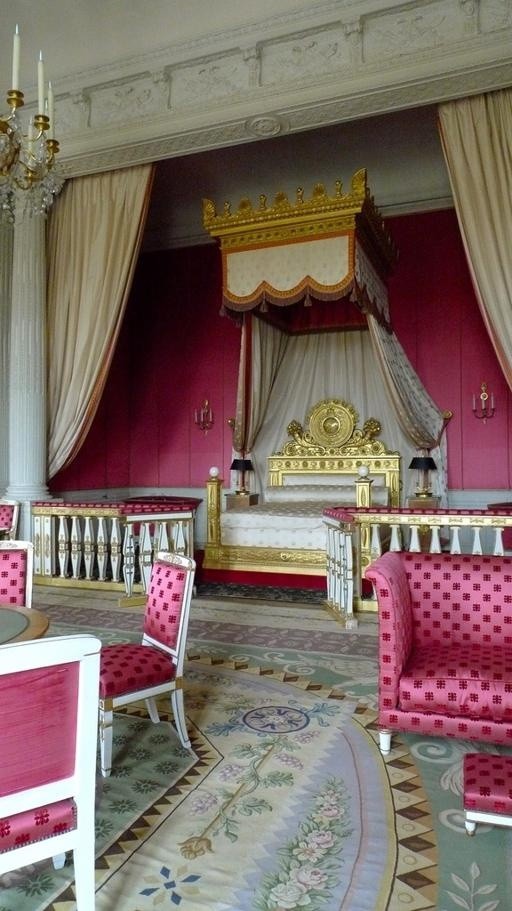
[200,398,406,579]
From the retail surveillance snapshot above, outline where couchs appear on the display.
[364,549,512,758]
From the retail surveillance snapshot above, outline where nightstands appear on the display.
[224,493,260,511]
[408,495,442,510]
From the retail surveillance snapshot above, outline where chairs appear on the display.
[99,551,199,779]
[1,631,105,910]
[0,500,21,540]
[0,538,36,610]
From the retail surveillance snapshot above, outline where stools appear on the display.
[460,752,512,838]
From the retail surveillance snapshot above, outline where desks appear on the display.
[0,605,51,645]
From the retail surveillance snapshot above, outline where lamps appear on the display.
[407,456,438,499]
[193,399,215,431]
[470,380,496,420]
[229,458,254,495]
